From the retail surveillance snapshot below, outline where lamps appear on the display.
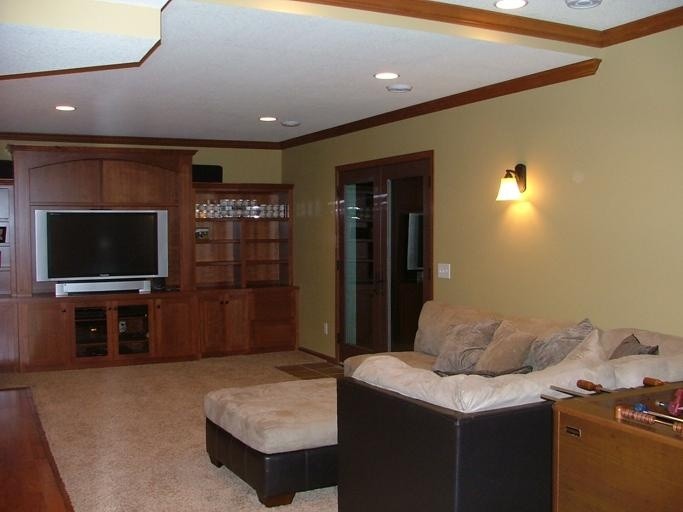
[495,163,527,202]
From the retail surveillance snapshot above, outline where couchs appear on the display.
[199,296,683,512]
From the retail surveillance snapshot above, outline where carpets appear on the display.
[2,350,337,511]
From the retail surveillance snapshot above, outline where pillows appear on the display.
[432,312,660,369]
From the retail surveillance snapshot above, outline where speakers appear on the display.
[192,164,223,183]
[0,160,14,178]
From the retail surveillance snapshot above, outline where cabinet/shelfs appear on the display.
[0,175,22,375]
[195,181,302,357]
[19,293,203,375]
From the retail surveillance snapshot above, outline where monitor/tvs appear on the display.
[35,209,169,298]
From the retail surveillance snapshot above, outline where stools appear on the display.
[204,376,337,506]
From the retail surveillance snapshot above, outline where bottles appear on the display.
[195,204,221,218]
